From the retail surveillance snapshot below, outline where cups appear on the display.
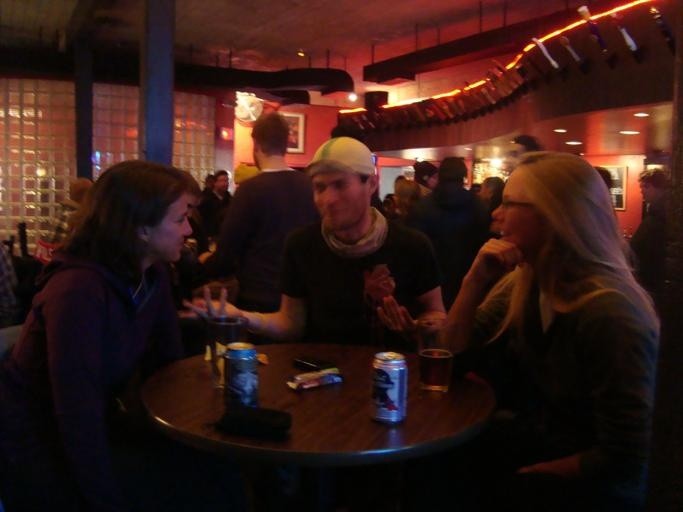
[416,319,453,394]
[208,315,249,390]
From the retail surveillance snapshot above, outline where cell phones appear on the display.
[292,355,337,370]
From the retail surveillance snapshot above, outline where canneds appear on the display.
[223,342,259,410]
[367,351,409,423]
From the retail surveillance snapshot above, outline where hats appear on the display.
[306,137,376,178]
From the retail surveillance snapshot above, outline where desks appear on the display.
[140,343,502,511]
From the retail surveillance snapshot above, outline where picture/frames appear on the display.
[278,110,305,154]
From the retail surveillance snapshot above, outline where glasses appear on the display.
[500,198,531,211]
